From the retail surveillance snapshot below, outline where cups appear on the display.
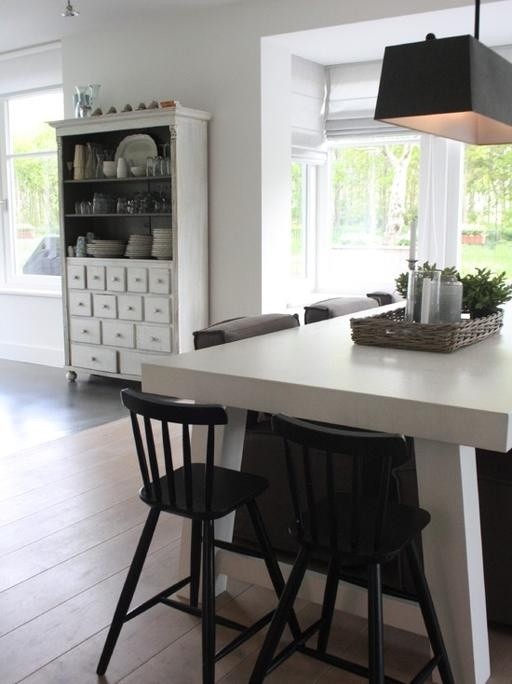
[73,145,84,168]
[162,156,171,175]
[67,245,74,256]
[74,201,80,215]
[117,173,127,178]
[73,168,84,180]
[74,236,85,257]
[406,270,440,325]
[153,156,162,175]
[145,156,154,176]
[79,200,92,214]
[116,158,127,173]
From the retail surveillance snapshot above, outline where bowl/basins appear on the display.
[130,166,145,176]
[102,160,116,177]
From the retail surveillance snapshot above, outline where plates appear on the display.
[124,234,151,259]
[114,133,158,174]
[151,228,172,260]
[86,239,124,257]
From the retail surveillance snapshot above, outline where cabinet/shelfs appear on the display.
[45,108,212,382]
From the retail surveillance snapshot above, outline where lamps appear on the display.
[375,34,512,146]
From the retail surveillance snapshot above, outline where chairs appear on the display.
[193,313,300,351]
[249,411,455,684]
[366,291,394,306]
[304,295,380,325]
[95,385,305,684]
